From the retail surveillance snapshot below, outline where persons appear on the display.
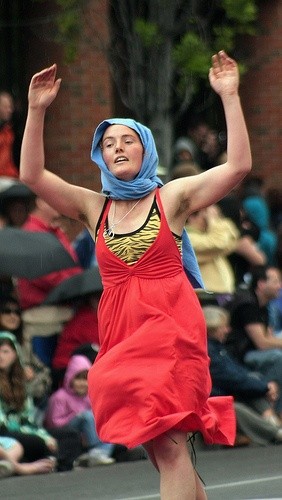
[20,47,254,499]
[1,93,282,478]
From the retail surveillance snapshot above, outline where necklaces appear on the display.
[102,198,141,238]
